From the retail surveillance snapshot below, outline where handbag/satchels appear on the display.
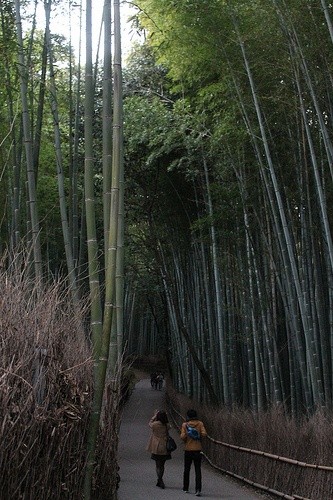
[186,425,201,440]
[165,436,177,452]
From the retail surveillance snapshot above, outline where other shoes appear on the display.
[182,487,189,493]
[194,489,201,495]
[156,479,165,489]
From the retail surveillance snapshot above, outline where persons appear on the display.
[179,409,207,496]
[150,370,163,391]
[147,409,172,489]
